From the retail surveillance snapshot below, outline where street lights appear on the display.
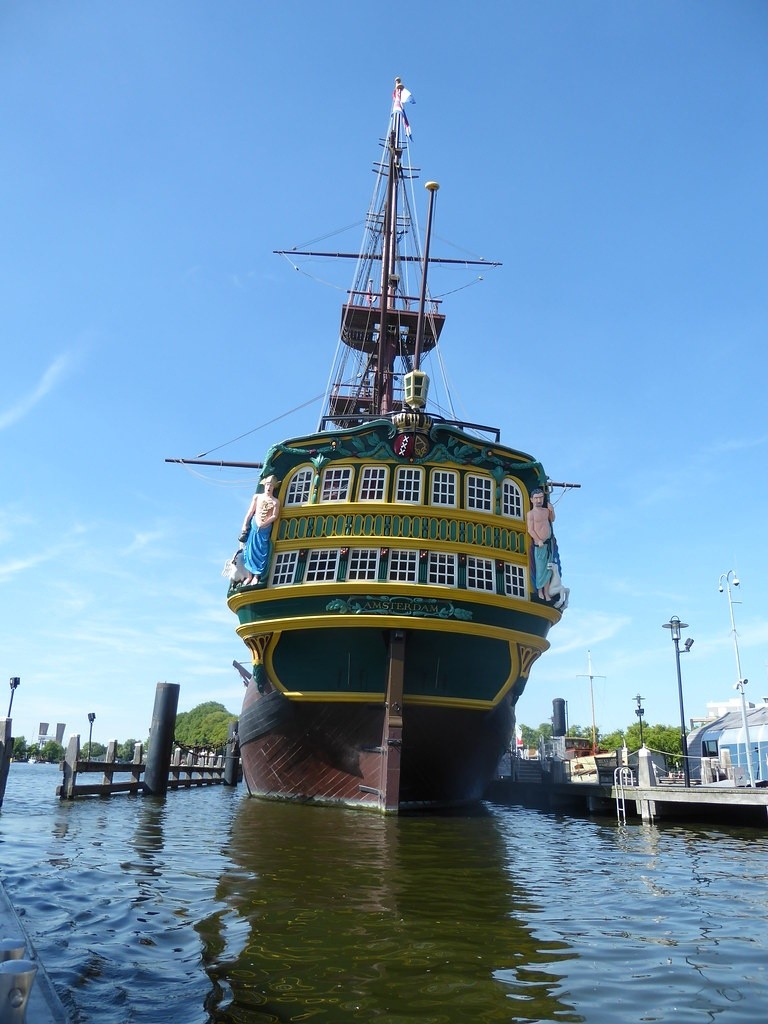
[87,712,96,761]
[7,677,21,718]
[633,693,646,746]
[717,569,757,789]
[662,616,696,789]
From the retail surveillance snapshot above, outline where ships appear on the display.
[162,78,584,817]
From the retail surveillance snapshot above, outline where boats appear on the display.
[28,757,37,764]
